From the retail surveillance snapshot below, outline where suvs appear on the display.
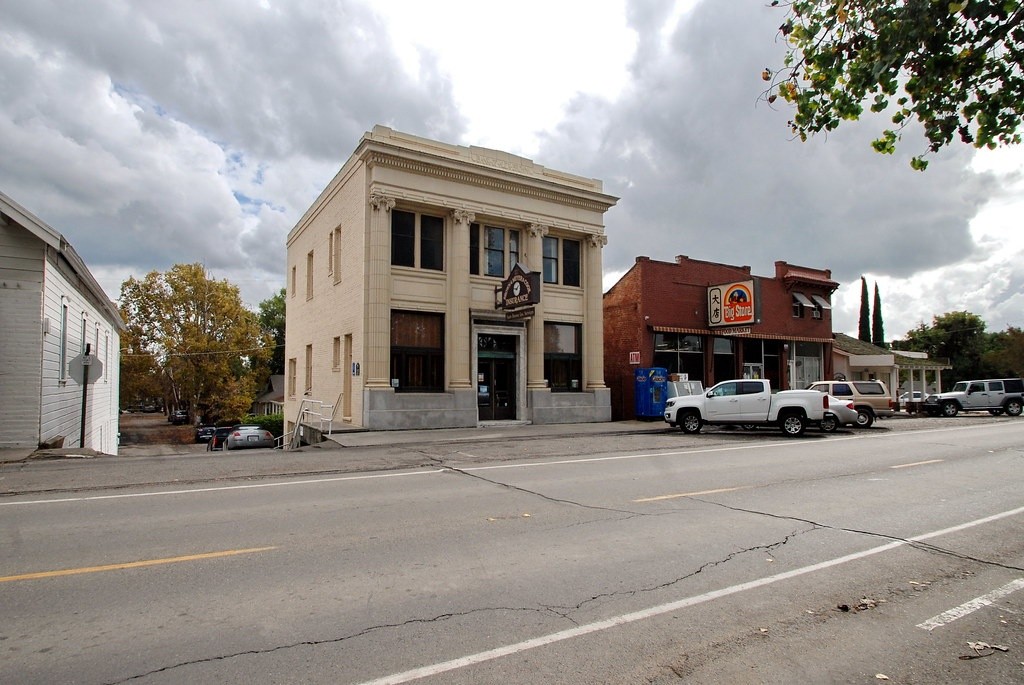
[804,379,894,430]
[207,427,231,452]
[175,410,189,424]
[194,424,216,444]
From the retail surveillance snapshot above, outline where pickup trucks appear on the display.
[926,378,1024,417]
[664,380,829,437]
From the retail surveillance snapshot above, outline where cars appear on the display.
[774,390,859,435]
[223,424,274,449]
[898,391,931,410]
